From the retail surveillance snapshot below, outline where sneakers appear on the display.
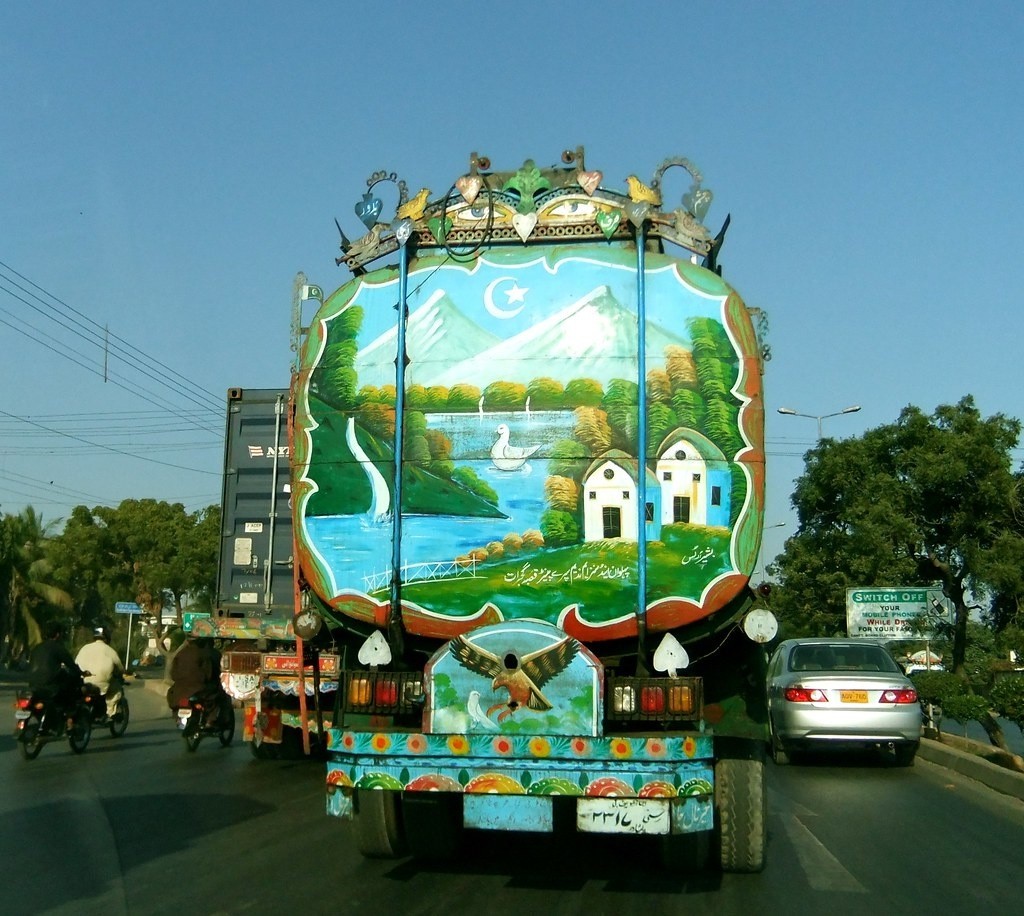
[205,722,221,732]
[66,725,86,736]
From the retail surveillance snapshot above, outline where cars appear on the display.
[765,637,921,765]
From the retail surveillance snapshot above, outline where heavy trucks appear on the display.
[287,138,772,874]
[193,388,408,762]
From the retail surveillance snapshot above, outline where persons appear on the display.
[72,624,128,725]
[166,634,223,730]
[25,623,90,730]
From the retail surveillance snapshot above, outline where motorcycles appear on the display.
[13,669,93,759]
[74,677,129,742]
[177,675,235,752]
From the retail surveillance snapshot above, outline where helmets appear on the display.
[94,627,111,638]
